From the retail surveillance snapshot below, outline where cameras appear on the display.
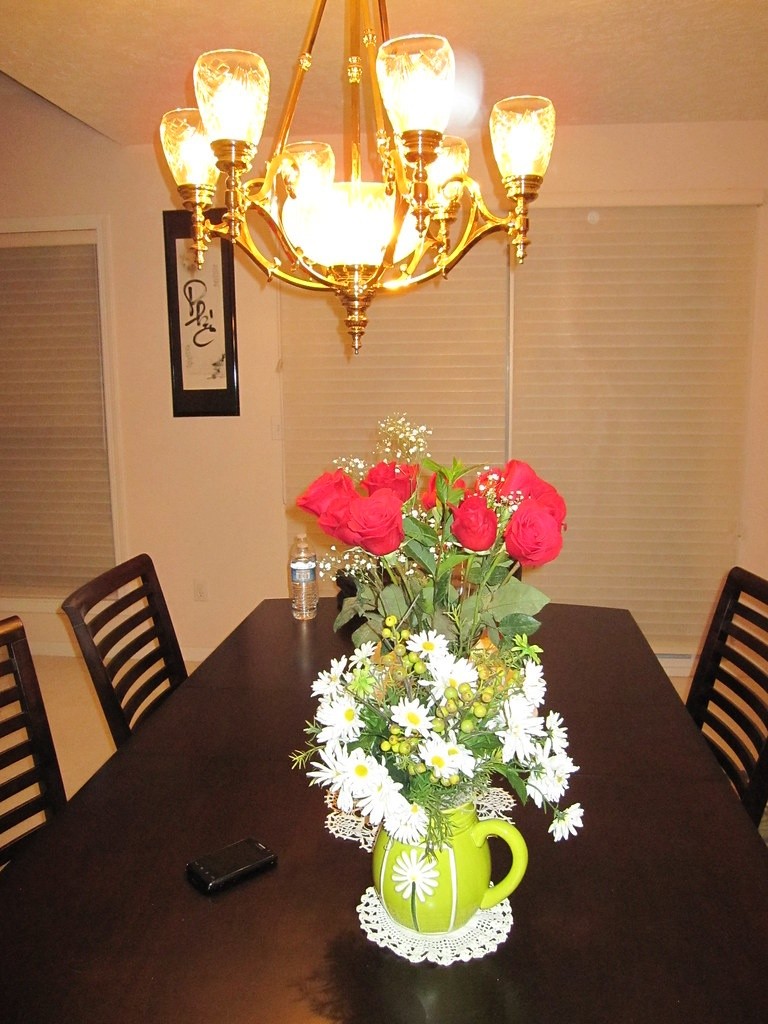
[186,837,278,896]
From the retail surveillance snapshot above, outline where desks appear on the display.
[0,597,768,1024]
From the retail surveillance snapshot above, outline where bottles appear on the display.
[292,543,317,620]
[291,534,318,604]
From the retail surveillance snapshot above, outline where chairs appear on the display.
[685,566,768,829]
[0,615,68,874]
[64,553,189,751]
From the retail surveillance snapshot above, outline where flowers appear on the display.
[296,624,586,861]
[297,418,569,662]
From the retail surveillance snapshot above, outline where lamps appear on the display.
[158,0,556,355]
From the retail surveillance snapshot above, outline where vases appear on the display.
[372,802,528,934]
